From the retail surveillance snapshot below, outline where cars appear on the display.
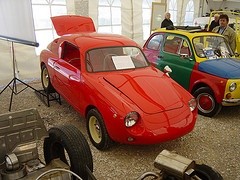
[135,25,240,117]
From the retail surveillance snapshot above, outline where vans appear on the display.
[194,17,240,61]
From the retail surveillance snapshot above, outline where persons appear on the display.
[161,13,173,28]
[204,14,236,57]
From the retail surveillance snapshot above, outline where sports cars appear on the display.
[39,15,199,151]
